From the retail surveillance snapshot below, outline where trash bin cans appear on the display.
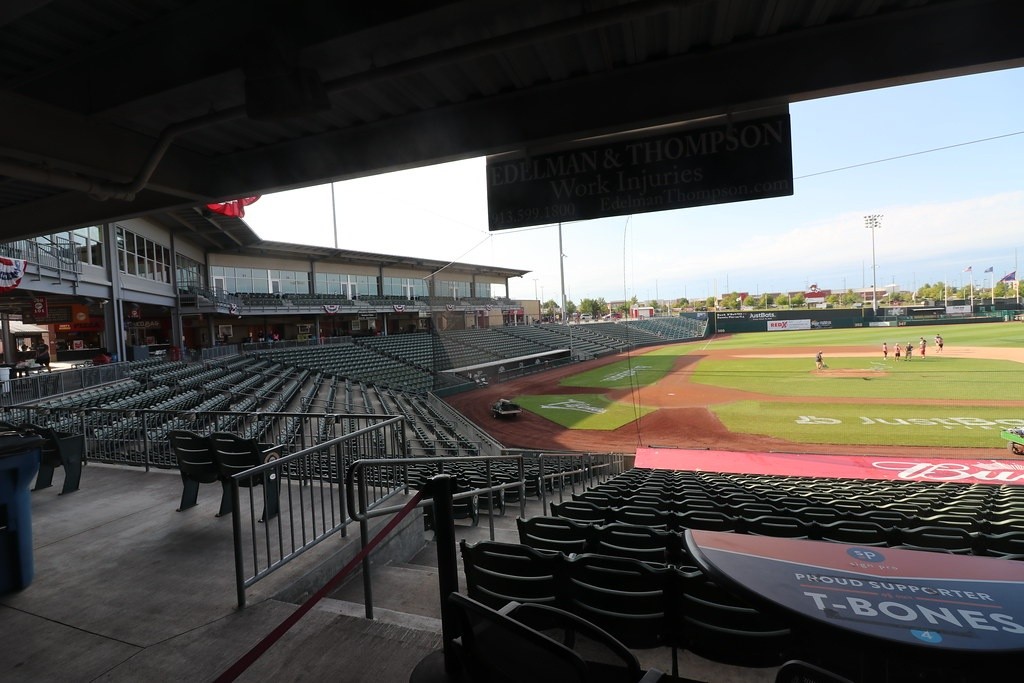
[0,422,45,597]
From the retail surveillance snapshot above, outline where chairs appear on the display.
[9,309,1024,683]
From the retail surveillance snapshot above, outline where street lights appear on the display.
[863,215,883,320]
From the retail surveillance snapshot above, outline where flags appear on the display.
[1000,271,1016,282]
[963,265,971,272]
[984,266,993,272]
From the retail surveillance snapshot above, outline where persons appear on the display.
[36,339,51,373]
[883,343,888,360]
[905,343,913,361]
[223,333,228,343]
[93,348,112,365]
[258,330,265,342]
[933,334,943,353]
[333,328,339,336]
[273,331,279,341]
[919,337,926,358]
[894,343,901,361]
[816,352,824,370]
